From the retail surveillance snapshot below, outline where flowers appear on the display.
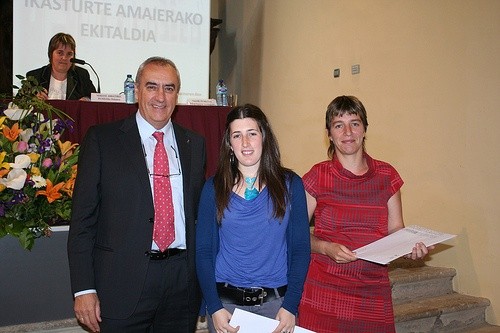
[0,74,81,252]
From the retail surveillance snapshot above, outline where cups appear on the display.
[227,94,237,107]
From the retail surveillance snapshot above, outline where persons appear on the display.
[15,33,97,101]
[67,56,207,333]
[196,103,311,333]
[298,95,435,333]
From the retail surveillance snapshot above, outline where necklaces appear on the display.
[243,174,259,200]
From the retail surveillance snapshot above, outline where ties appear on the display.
[151,131,175,252]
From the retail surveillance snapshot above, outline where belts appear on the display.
[219,282,287,307]
[149,248,182,260]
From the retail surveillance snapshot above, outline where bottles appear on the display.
[124,74,134,104]
[216,79,227,106]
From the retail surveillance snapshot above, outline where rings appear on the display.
[79,317,84,321]
[281,331,290,333]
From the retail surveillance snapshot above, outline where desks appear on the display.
[0,98,240,181]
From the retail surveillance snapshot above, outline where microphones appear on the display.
[69,58,99,92]
